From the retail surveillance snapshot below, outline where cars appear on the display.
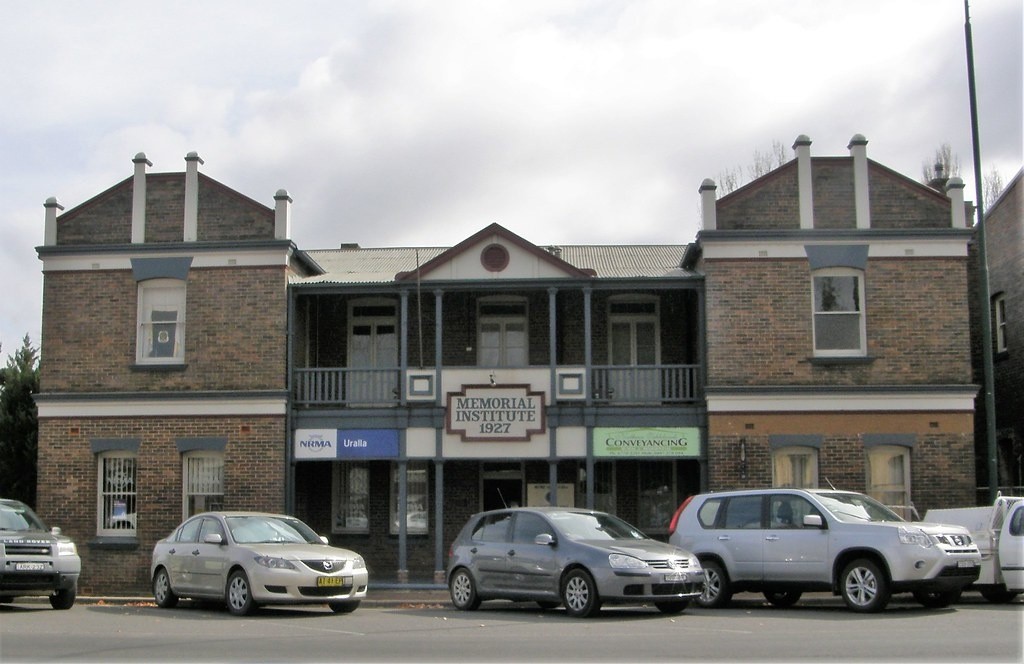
[148,510,370,615]
[443,507,706,618]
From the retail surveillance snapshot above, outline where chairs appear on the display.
[776,501,799,528]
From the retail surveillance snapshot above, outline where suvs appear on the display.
[0,498,81,609]
[666,486,984,613]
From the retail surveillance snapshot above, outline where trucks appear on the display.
[920,493,1024,603]
